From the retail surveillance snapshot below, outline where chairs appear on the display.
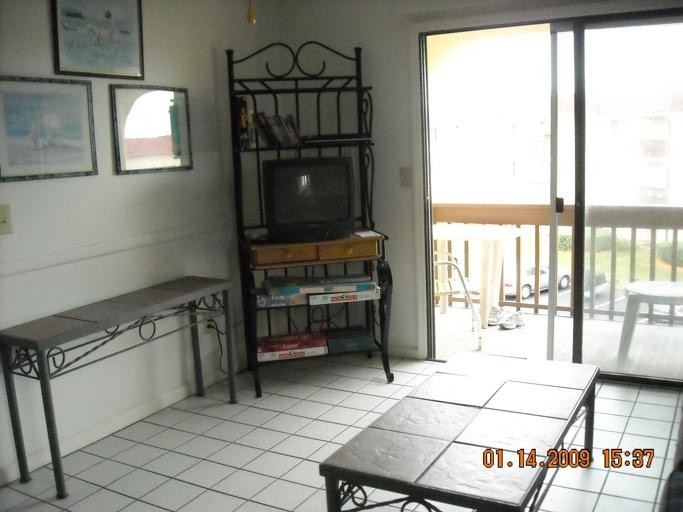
[434,252,482,350]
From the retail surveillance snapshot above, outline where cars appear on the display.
[504,266,569,298]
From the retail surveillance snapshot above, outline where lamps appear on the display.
[246,0,257,25]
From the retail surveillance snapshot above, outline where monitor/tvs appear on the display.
[261,155,356,245]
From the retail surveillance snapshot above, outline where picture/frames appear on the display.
[0,75,99,183]
[51,0,145,81]
[110,84,194,176]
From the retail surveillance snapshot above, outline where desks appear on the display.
[0,275,238,500]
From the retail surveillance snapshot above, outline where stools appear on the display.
[617,281,683,366]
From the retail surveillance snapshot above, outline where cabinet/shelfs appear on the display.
[224,39,395,399]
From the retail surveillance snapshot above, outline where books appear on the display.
[238,97,302,149]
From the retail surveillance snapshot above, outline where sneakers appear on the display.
[487,305,502,326]
[500,311,525,330]
